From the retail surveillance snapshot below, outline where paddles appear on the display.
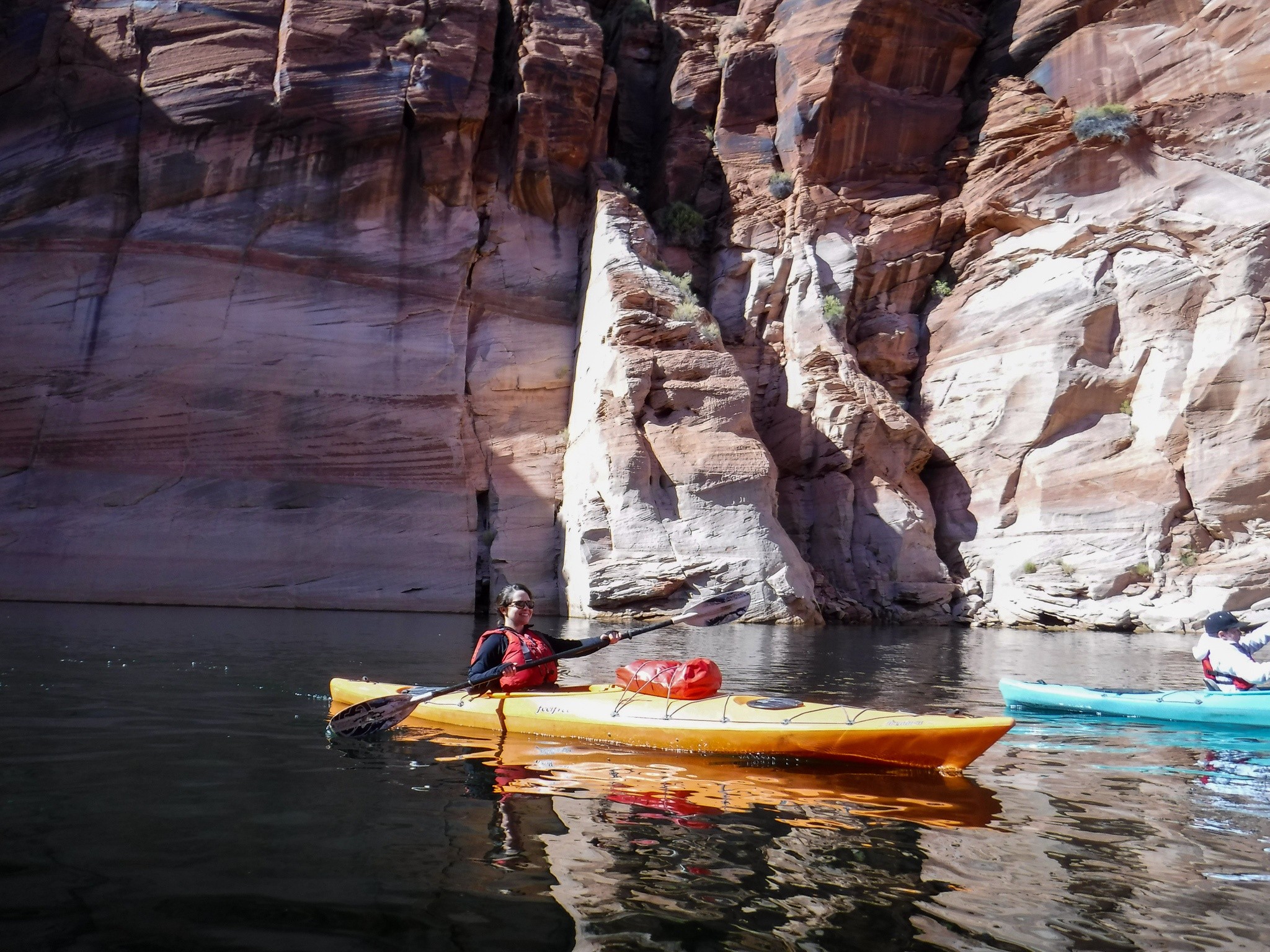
[330,586,752,735]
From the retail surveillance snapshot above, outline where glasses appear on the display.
[511,600,535,609]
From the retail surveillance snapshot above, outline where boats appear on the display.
[998,676,1270,730]
[325,672,1019,773]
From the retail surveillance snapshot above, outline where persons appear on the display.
[1192,610,1270,692]
[467,583,622,696]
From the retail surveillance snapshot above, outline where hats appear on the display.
[1204,610,1252,633]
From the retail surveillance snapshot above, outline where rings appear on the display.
[502,670,505,672]
[511,665,516,668]
